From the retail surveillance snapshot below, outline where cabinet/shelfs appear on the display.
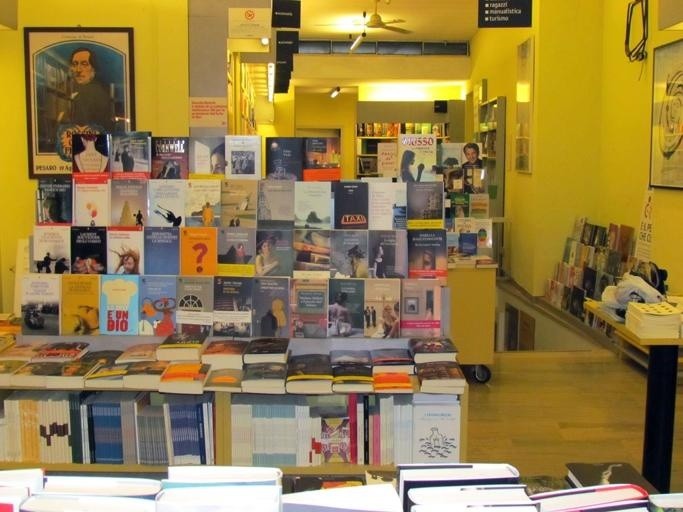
[355,95,505,277]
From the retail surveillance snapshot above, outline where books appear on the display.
[1,133,683,512]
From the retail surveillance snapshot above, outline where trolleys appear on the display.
[447,253,499,384]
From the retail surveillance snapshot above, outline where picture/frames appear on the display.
[649,39,683,191]
[23,27,136,180]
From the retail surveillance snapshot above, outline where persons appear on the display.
[56,46,112,132]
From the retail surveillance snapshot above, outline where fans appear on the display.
[316,0,414,39]
